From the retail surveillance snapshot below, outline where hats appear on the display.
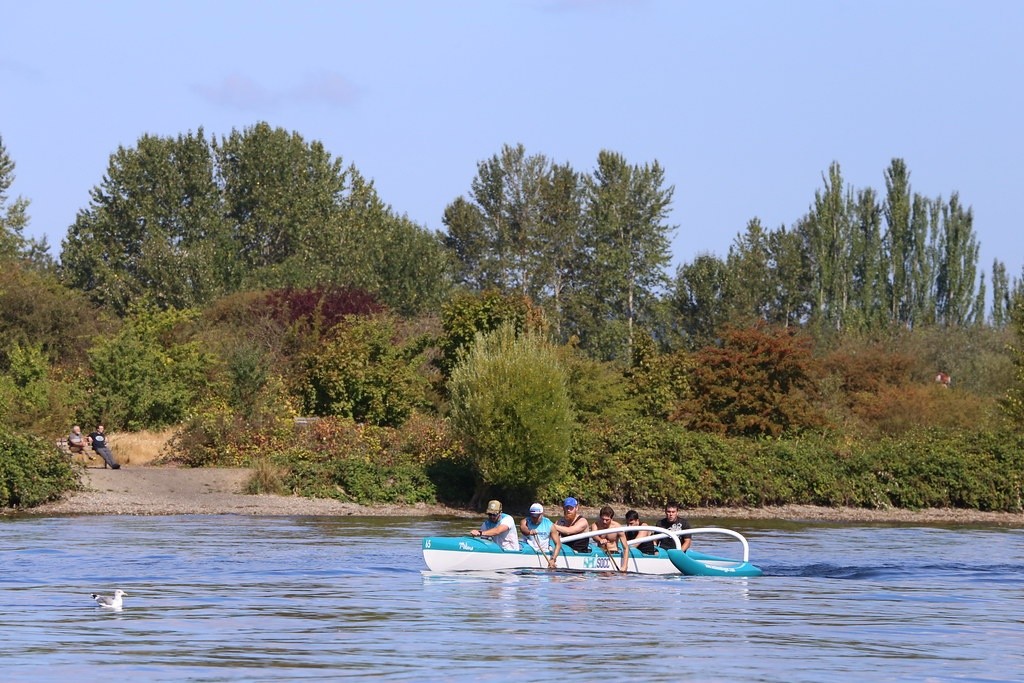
[529,503,543,515]
[563,497,578,507]
[485,500,502,514]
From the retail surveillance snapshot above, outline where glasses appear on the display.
[488,512,499,517]
[530,514,541,518]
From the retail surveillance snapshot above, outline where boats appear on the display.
[422,526,763,577]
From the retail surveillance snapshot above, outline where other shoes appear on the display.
[112,463,120,469]
[89,456,96,461]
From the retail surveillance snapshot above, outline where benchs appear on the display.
[56,438,107,469]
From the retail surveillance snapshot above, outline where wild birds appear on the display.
[91,589,128,608]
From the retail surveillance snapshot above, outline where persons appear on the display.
[471,500,519,551]
[67,426,96,461]
[554,497,589,553]
[625,510,655,555]
[591,506,629,571]
[518,503,561,569]
[653,501,692,553]
[87,425,121,469]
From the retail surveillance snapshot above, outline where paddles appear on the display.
[604,543,621,573]
[534,534,551,566]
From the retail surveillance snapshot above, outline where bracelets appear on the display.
[551,558,556,562]
[478,531,482,536]
